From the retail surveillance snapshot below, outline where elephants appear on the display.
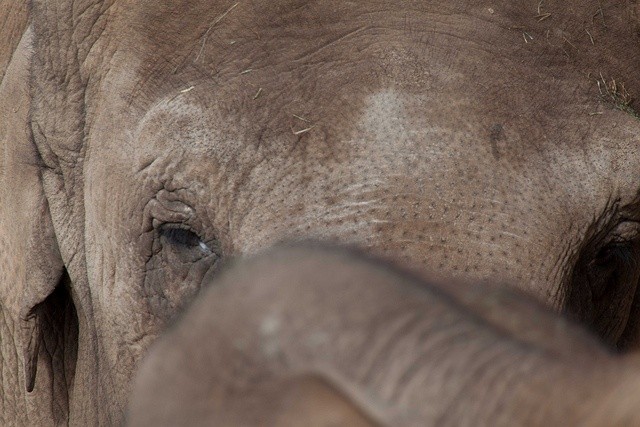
[0,0,640,427]
